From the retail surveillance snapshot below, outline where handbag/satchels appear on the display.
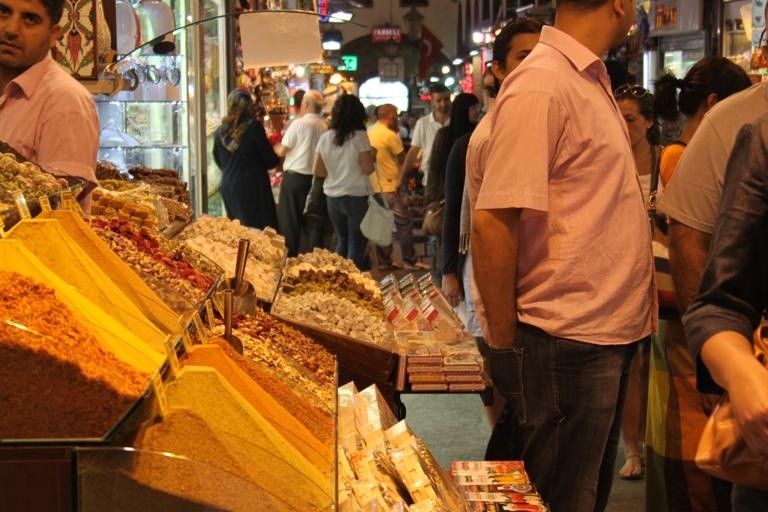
[646,145,677,310]
[422,200,444,237]
[359,163,394,247]
[750,30,768,69]
[694,326,768,493]
[303,175,329,218]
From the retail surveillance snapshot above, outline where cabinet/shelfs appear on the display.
[159,206,496,420]
[1,140,552,512]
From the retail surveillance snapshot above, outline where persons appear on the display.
[394,84,451,270]
[467,1,658,512]
[648,75,689,148]
[212,88,281,234]
[643,53,755,511]
[440,134,470,308]
[659,77,767,511]
[367,103,422,272]
[397,111,416,148]
[311,93,377,272]
[425,92,480,204]
[1,1,100,215]
[681,114,768,511]
[462,17,542,432]
[615,85,659,479]
[272,89,329,258]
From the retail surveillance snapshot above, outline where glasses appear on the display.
[614,85,649,99]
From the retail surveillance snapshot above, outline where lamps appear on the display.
[328,3,353,23]
[397,0,433,41]
[321,25,342,49]
[112,10,324,69]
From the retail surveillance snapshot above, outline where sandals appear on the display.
[619,455,641,479]
